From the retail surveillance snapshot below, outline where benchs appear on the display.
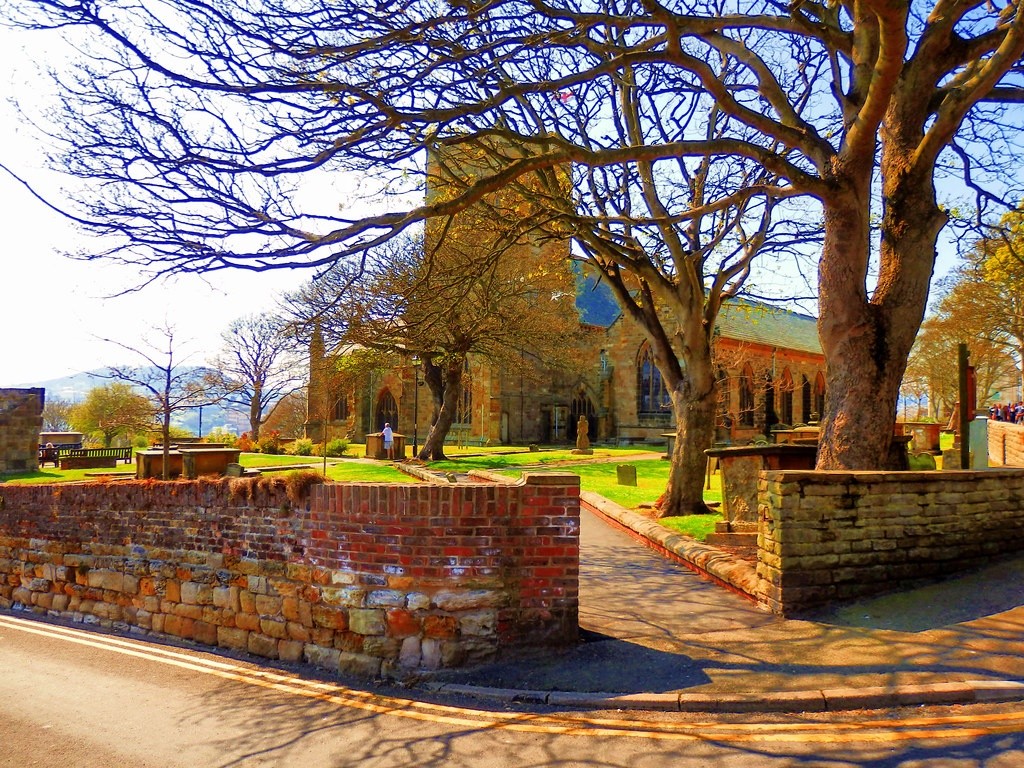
[39,442,84,464]
[37,446,60,468]
[69,446,132,465]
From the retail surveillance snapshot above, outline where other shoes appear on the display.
[392,457,395,459]
[384,457,390,459]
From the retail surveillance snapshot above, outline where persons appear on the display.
[39,443,55,465]
[380,423,395,460]
[577,415,589,449]
[989,401,1024,425]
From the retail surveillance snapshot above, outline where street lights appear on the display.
[410,355,422,457]
